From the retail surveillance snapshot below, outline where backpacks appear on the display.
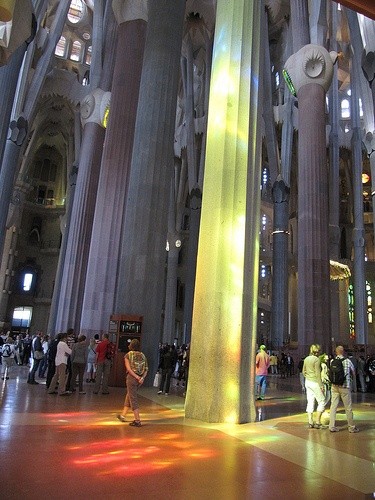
[328,356,348,388]
[2,344,13,358]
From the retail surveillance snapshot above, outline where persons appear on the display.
[0,330,35,368]
[116,338,148,427]
[254,345,271,400]
[347,353,375,393]
[298,356,306,392]
[1,337,22,379]
[87,334,116,386]
[69,334,86,394]
[48,333,71,396]
[328,345,360,433]
[93,333,112,394]
[38,329,79,391]
[301,343,329,429]
[266,352,294,379]
[156,338,191,396]
[27,329,44,384]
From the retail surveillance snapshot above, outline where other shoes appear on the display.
[157,390,162,395]
[67,379,111,395]
[164,393,168,396]
[47,391,58,394]
[116,414,126,422]
[59,392,71,396]
[307,422,314,429]
[128,420,142,428]
[27,378,39,385]
[314,423,328,429]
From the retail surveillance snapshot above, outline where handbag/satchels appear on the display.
[34,351,43,360]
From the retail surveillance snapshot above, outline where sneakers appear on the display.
[329,427,339,432]
[348,424,359,433]
